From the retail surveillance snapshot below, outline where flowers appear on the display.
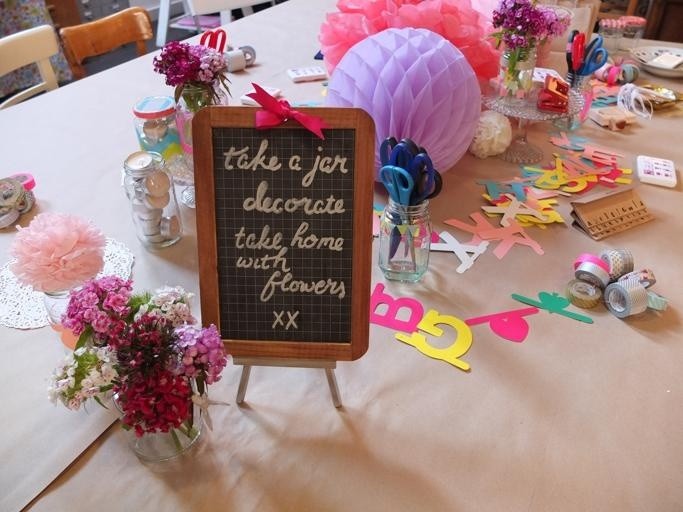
[9,211,105,291]
[152,39,233,110]
[488,1,574,93]
[47,276,226,434]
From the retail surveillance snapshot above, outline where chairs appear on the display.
[1,6,154,109]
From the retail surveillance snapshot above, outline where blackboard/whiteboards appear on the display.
[192,105,371,359]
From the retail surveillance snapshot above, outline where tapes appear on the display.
[224,43,256,72]
[566,246,669,318]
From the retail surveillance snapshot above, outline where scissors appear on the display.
[567,28,608,87]
[198,30,227,55]
[378,135,442,273]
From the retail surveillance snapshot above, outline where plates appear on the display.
[631,46,683,78]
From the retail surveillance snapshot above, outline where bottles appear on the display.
[554,73,594,133]
[131,96,183,151]
[495,47,540,107]
[121,150,183,249]
[377,197,432,283]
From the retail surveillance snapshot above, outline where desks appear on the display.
[1,0,683,512]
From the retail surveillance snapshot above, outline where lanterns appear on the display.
[325,25,482,186]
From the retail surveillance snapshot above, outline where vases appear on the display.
[499,45,536,106]
[173,81,231,174]
[110,386,209,460]
[41,285,95,351]
